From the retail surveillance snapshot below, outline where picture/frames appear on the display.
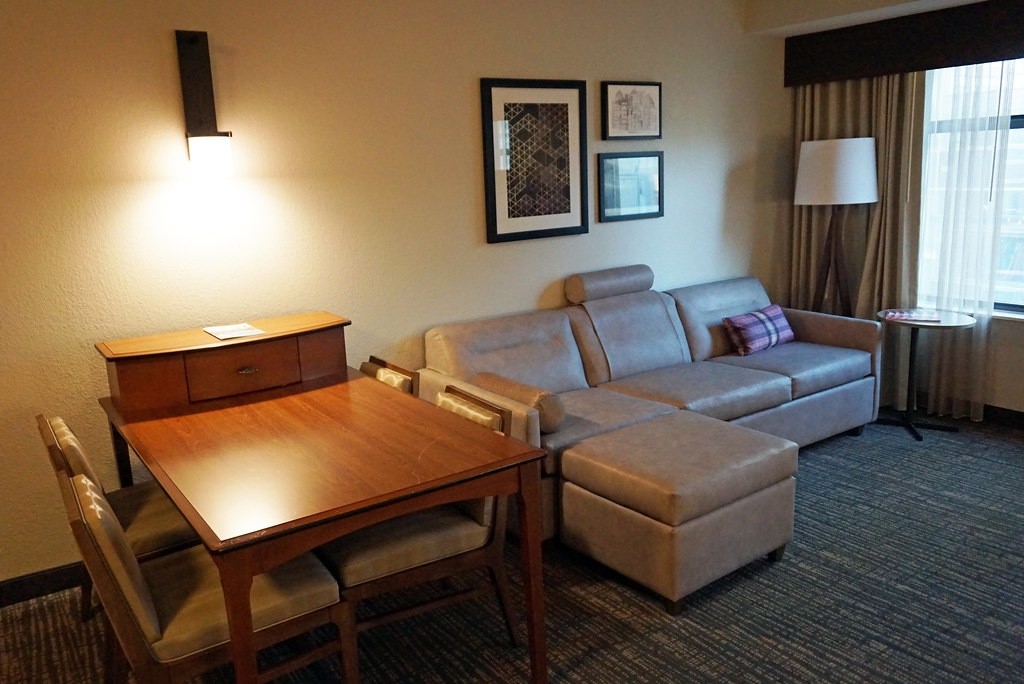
[600,82,662,140]
[480,78,588,244]
[599,151,664,222]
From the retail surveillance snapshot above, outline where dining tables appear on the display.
[98,365,548,684]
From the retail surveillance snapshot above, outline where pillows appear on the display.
[472,372,564,434]
[722,304,794,356]
[565,264,654,303]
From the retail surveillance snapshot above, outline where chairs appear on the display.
[315,384,521,648]
[56,470,359,684]
[360,355,419,397]
[36,413,201,622]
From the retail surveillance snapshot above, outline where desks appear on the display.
[875,308,977,439]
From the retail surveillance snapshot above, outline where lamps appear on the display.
[794,137,879,317]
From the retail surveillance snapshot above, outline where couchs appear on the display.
[417,277,882,616]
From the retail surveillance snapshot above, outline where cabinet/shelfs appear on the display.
[94,311,352,412]
